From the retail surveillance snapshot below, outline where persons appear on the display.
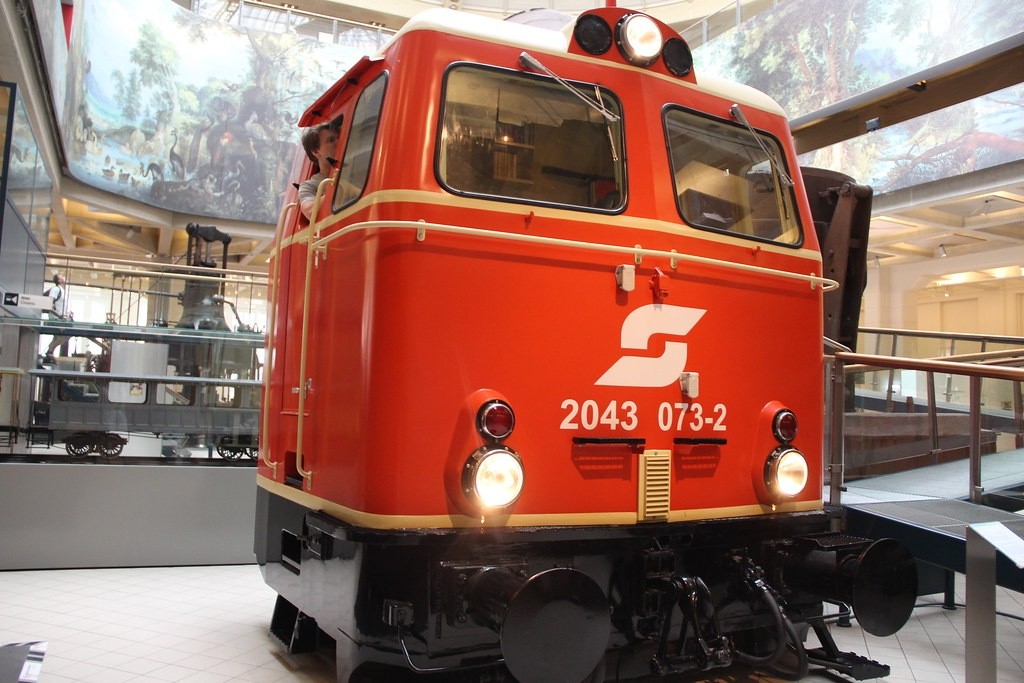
[44,273,75,363]
[296,122,362,222]
